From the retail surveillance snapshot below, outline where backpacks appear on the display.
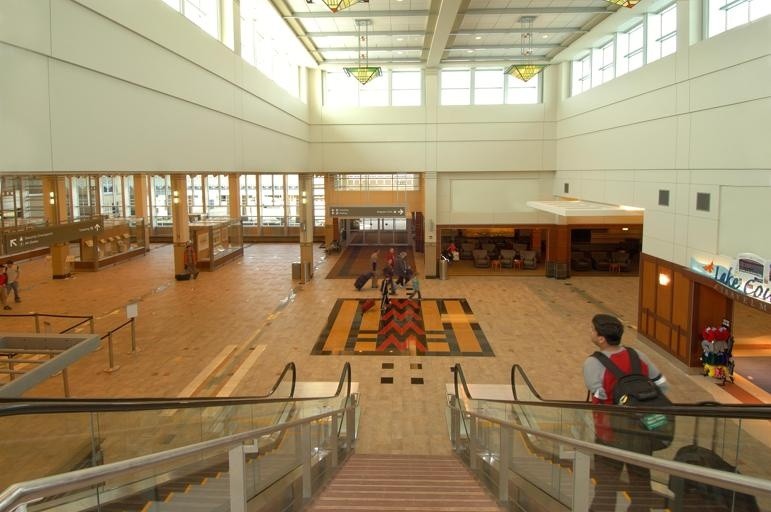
[592,346,675,453]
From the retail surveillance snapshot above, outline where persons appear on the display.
[584,313,670,512]
[371,247,422,308]
[5,260,22,302]
[184,245,199,279]
[0,265,12,309]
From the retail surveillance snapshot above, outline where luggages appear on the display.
[666,402,759,511]
[354,272,371,290]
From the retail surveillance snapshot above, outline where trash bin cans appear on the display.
[439,260,449,280]
[546,263,567,279]
[292,262,301,279]
[301,261,311,281]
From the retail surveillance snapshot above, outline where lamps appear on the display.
[342,17,384,85]
[319,0,368,13]
[605,1,646,10]
[502,14,546,84]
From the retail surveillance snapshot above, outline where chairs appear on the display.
[571,248,632,274]
[457,233,538,276]
